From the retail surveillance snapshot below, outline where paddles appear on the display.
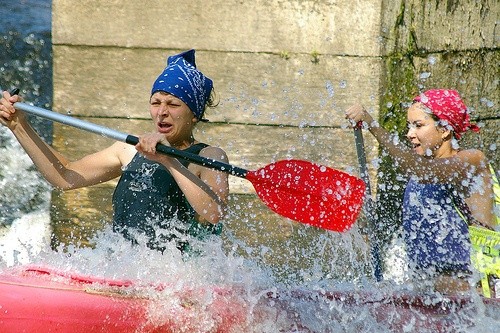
[6,89,366,233]
[353,113,384,281]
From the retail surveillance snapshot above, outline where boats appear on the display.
[2,230,500,333]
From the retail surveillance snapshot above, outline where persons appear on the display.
[0,49,229,262]
[345,88,500,298]
